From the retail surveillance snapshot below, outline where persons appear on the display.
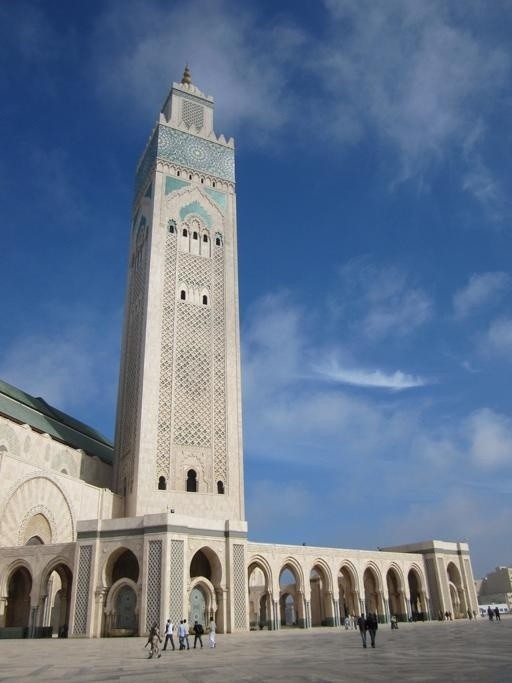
[177,620,186,650]
[193,621,203,649]
[162,619,175,651]
[438,608,477,623]
[487,605,493,621]
[183,619,190,650]
[367,612,378,649]
[494,607,500,621]
[357,614,368,648]
[391,614,396,629]
[344,614,360,630]
[206,616,216,648]
[144,622,163,659]
